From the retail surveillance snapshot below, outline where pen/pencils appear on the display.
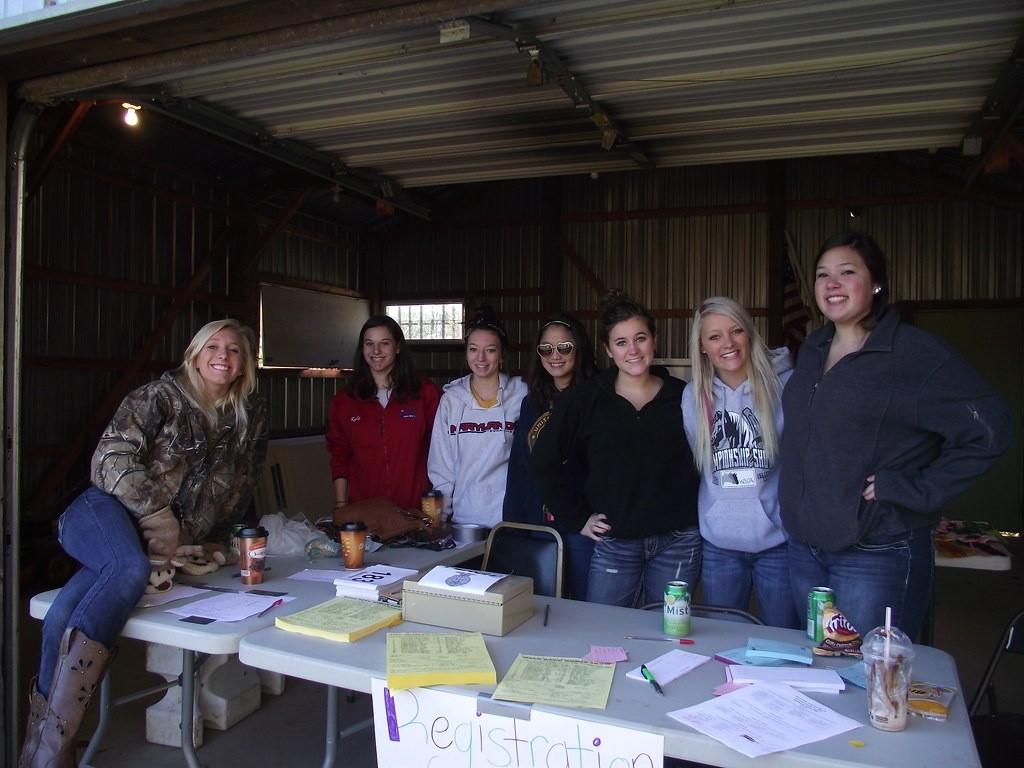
[543,604,549,626]
[623,635,694,645]
[232,567,271,578]
[640,664,665,696]
[258,599,282,619]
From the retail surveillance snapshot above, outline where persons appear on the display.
[681,296,796,627]
[326,316,444,510]
[780,232,1014,643]
[427,314,530,529]
[502,314,599,601]
[20,319,267,768]
[529,288,703,610]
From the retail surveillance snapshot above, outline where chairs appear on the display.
[968,614,1024,767]
[483,523,564,600]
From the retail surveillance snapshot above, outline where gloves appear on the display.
[137,506,178,568]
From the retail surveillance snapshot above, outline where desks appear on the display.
[29,519,1010,768]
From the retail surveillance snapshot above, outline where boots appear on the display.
[19,623,119,768]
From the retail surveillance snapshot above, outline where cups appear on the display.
[339,521,368,571]
[236,526,269,586]
[860,626,917,732]
[421,490,443,528]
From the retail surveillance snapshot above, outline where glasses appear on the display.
[537,341,578,360]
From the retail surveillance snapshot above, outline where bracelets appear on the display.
[336,501,346,503]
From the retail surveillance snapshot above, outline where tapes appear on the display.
[451,522,487,542]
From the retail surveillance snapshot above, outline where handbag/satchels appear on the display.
[333,499,436,543]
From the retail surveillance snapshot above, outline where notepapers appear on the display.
[745,637,813,665]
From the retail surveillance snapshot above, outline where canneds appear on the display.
[664,580,692,637]
[229,523,248,564]
[806,587,836,641]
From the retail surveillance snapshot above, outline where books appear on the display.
[386,632,497,689]
[275,596,402,644]
[334,564,419,600]
[626,637,846,694]
